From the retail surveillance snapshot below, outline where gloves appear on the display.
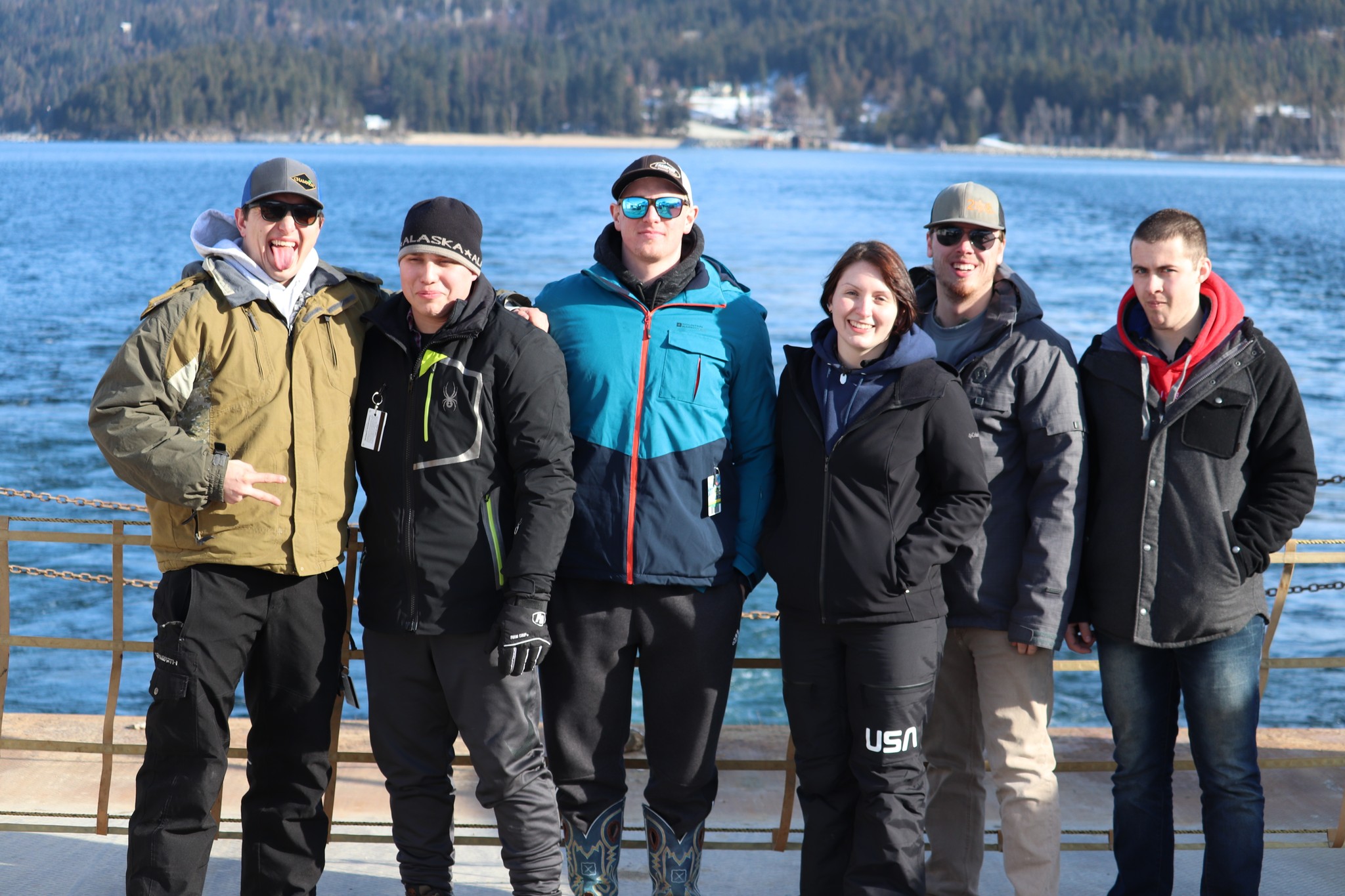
[482,595,552,676]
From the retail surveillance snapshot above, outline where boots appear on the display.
[559,796,627,896]
[641,801,707,896]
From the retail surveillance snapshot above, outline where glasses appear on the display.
[931,228,994,251]
[245,199,321,226]
[617,196,685,219]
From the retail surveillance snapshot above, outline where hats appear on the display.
[612,155,692,206]
[923,181,1005,232]
[240,157,325,212]
[398,196,484,278]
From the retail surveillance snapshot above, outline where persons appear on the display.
[1064,208,1318,896]
[350,196,578,896]
[531,154,776,896]
[89,157,549,896]
[765,241,992,896]
[908,181,1089,896]
